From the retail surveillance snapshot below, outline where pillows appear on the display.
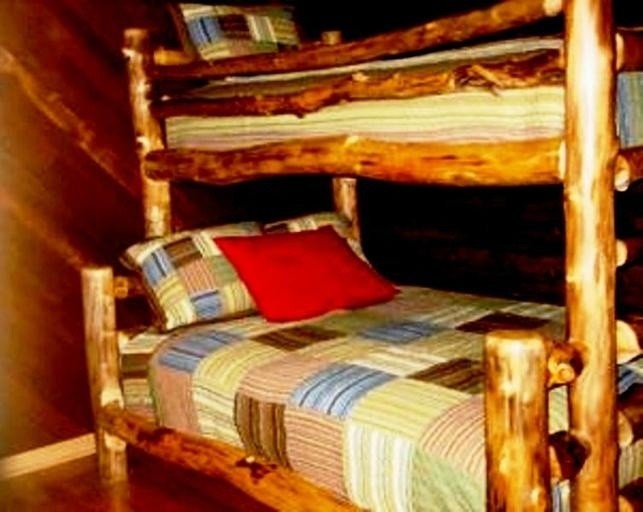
[118,211,401,333]
[164,2,303,61]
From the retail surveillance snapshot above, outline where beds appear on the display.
[122,0,643,512]
[80,265,643,512]
